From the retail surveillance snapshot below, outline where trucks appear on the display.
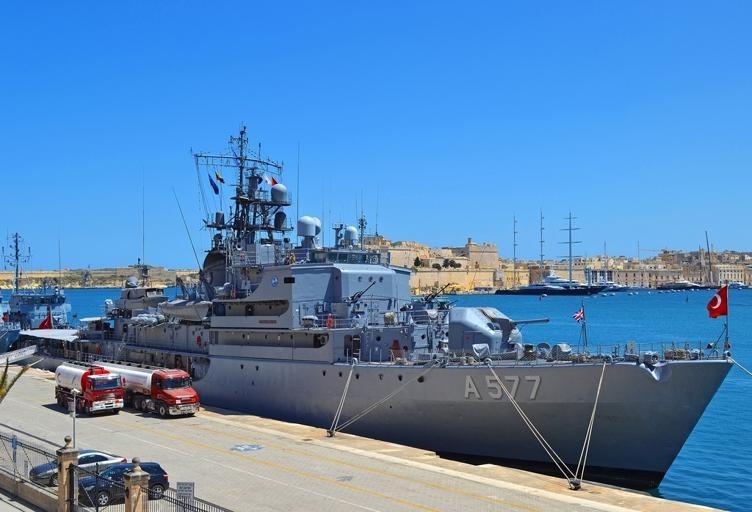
[54,358,200,418]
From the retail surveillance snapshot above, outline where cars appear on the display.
[29,449,128,488]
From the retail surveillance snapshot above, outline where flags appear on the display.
[215,169,224,183]
[272,176,277,186]
[208,173,219,195]
[573,308,583,323]
[38,313,51,329]
[265,174,270,184]
[256,174,263,185]
[706,285,728,318]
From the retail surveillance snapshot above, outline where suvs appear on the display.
[78,462,170,507]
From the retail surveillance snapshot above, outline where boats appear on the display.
[656,280,749,290]
[0,283,72,333]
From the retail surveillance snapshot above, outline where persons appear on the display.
[1,309,33,330]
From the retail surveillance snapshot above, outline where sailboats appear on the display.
[495,210,631,296]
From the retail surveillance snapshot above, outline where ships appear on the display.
[26,122,736,491]
